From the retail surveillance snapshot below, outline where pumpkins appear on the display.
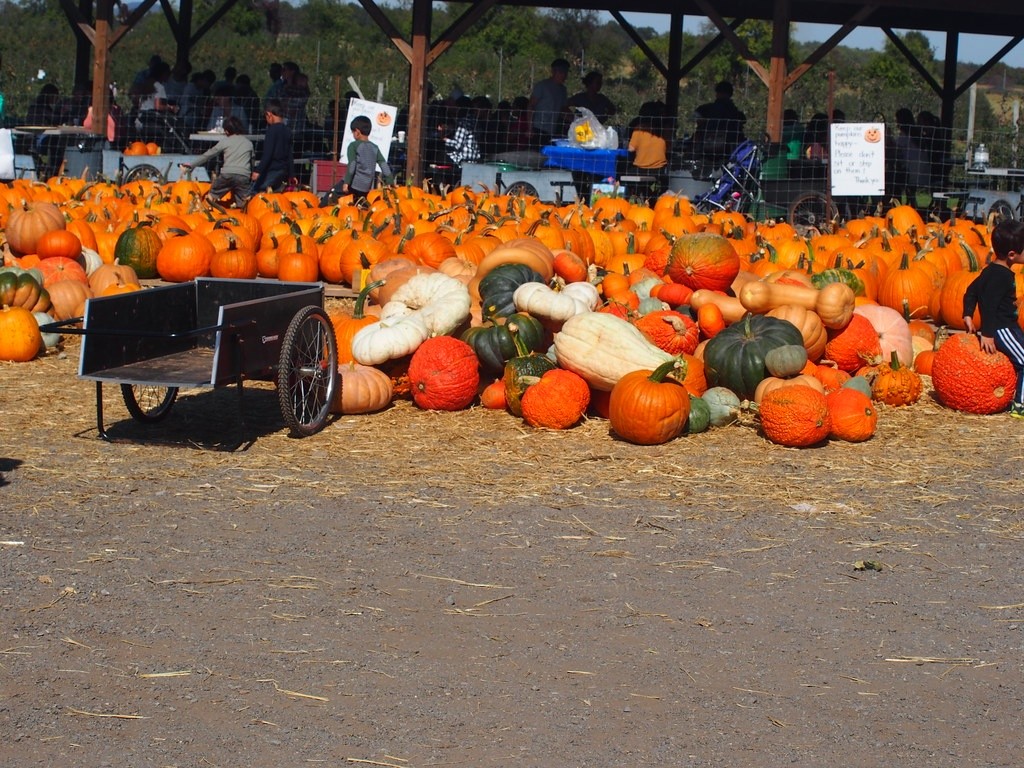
[0,161,1024,448]
[123,140,160,156]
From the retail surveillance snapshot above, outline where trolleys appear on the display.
[758,178,841,231]
[103,151,211,187]
[460,162,577,205]
[36,276,340,443]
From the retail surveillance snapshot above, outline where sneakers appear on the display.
[1010,399,1024,418]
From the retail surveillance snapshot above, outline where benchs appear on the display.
[619,175,656,198]
[549,181,589,206]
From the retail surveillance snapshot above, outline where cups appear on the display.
[398,130,405,144]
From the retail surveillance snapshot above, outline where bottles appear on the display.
[974,143,989,171]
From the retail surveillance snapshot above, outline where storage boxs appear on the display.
[590,183,626,208]
[313,159,348,193]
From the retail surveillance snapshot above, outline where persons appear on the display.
[963,220,1024,419]
[28,54,941,218]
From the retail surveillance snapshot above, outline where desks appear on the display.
[254,158,309,192]
[189,134,266,175]
[541,143,630,209]
[13,125,59,183]
[968,168,1024,223]
